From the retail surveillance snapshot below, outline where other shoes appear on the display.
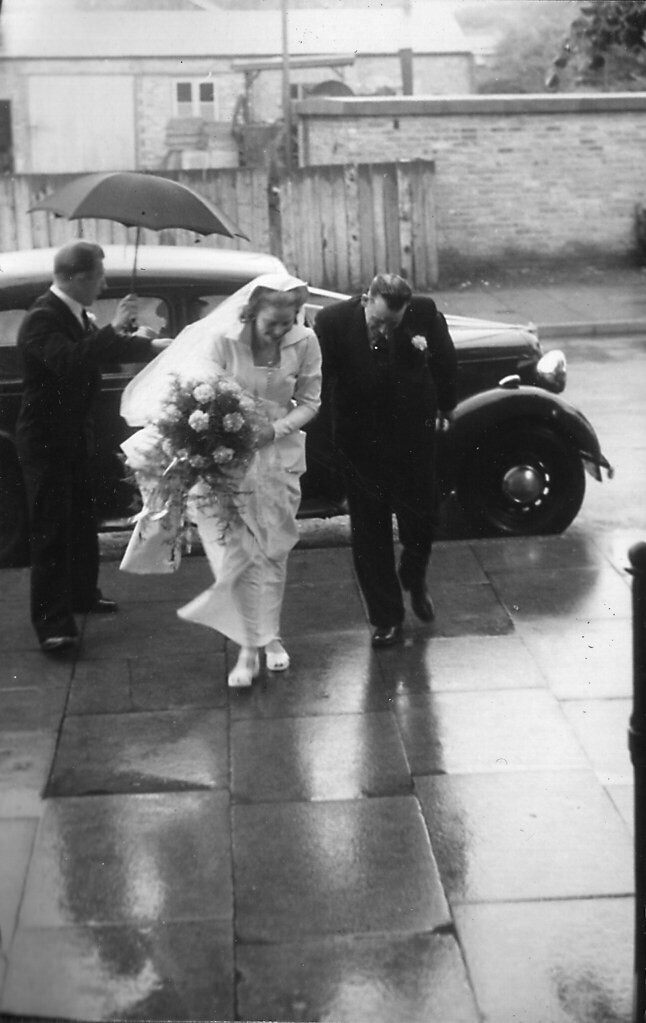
[40,630,80,651]
[69,599,118,613]
[227,652,260,688]
[262,645,290,672]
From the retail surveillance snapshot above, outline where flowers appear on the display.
[154,374,267,470]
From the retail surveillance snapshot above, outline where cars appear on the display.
[0,245,616,572]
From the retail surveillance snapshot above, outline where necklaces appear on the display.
[268,361,272,365]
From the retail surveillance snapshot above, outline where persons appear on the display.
[17,240,173,651]
[120,273,321,688]
[316,272,460,647]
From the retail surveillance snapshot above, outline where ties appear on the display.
[373,336,389,372]
[81,308,91,332]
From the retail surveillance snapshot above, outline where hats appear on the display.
[155,295,209,318]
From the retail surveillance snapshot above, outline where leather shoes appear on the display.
[371,624,403,647]
[395,559,437,621]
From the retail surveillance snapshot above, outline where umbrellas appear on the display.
[25,173,250,333]
